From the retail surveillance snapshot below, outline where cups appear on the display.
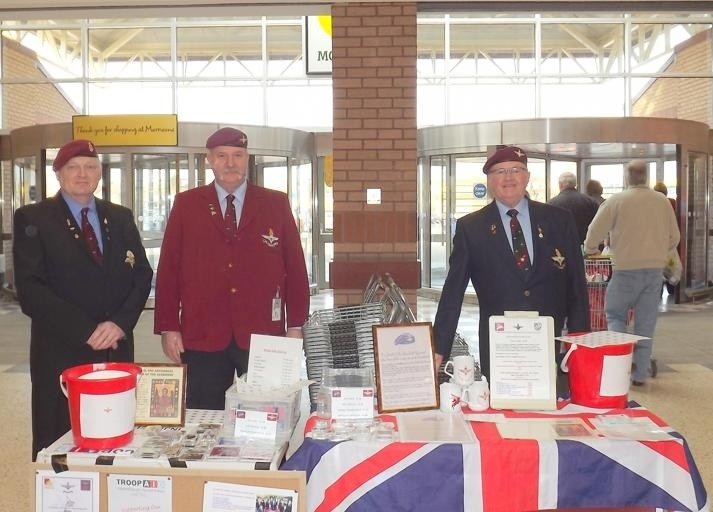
[439,355,490,413]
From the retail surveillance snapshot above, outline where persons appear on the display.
[432,144,593,400]
[547,170,599,247]
[585,177,607,206]
[155,388,173,417]
[255,495,292,511]
[580,160,682,387]
[653,182,677,300]
[11,137,152,464]
[153,126,312,410]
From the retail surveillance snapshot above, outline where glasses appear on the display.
[487,167,529,177]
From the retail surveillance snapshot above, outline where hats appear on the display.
[482,146,527,176]
[204,126,247,151]
[51,139,98,173]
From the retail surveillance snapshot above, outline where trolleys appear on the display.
[305,274,473,417]
[559,248,638,357]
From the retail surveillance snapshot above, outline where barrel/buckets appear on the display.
[559,331,638,409]
[58,361,144,452]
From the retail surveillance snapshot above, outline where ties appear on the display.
[76,207,104,268]
[504,208,532,271]
[221,193,239,240]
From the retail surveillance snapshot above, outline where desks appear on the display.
[34,409,301,512]
[286,395,706,512]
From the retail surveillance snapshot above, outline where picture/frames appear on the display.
[305,16,333,75]
[134,362,188,428]
[372,322,439,414]
